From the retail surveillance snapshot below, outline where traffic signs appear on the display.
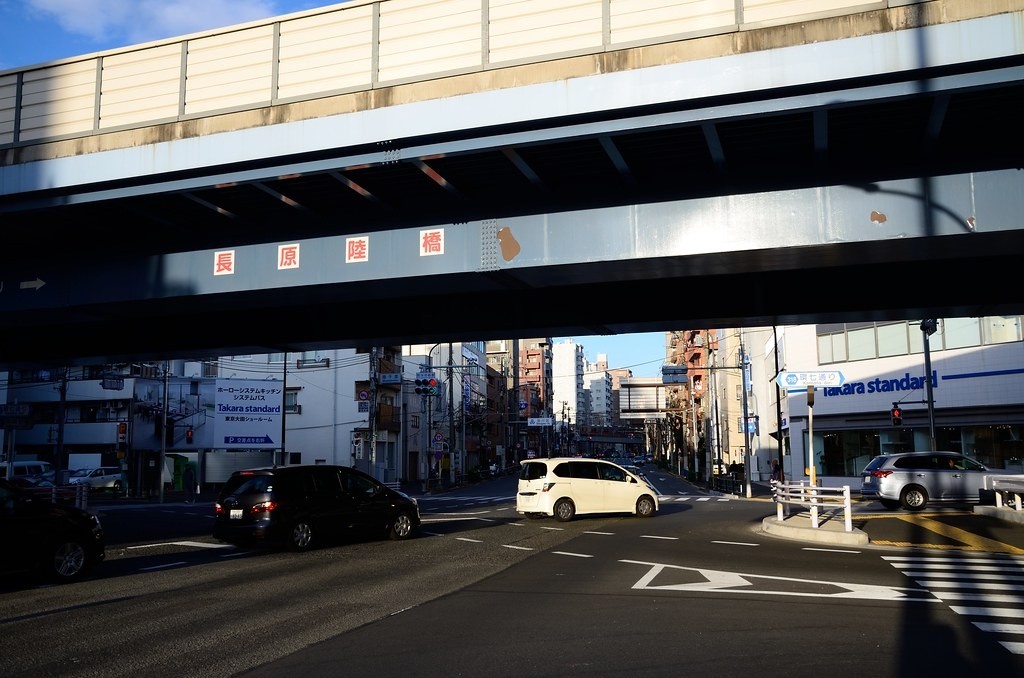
[774,370,846,388]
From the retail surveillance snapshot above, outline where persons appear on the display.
[946,458,959,470]
[771,459,780,481]
[182,464,196,504]
[729,461,739,480]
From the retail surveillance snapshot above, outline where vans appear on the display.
[515,456,659,522]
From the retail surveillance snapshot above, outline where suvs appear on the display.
[859,449,1024,510]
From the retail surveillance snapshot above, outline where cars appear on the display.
[210,463,423,553]
[0,460,123,496]
[0,476,106,582]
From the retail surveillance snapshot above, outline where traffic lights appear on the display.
[414,377,437,395]
[890,408,903,427]
